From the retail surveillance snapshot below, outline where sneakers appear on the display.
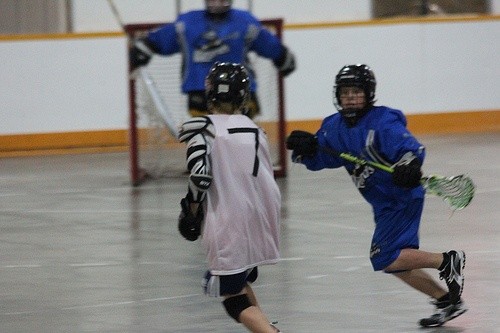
[420,298,467,327]
[440,250,466,302]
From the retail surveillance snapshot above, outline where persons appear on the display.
[284,64,469,327]
[131,0,296,119]
[178,62,282,333]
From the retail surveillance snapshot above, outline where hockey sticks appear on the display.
[285,136,475,211]
[108,0,255,142]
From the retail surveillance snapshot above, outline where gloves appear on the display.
[134,35,153,65]
[276,47,295,76]
[393,159,421,188]
[179,195,204,241]
[286,130,319,160]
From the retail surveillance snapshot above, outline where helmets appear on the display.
[332,64,376,127]
[204,62,248,112]
